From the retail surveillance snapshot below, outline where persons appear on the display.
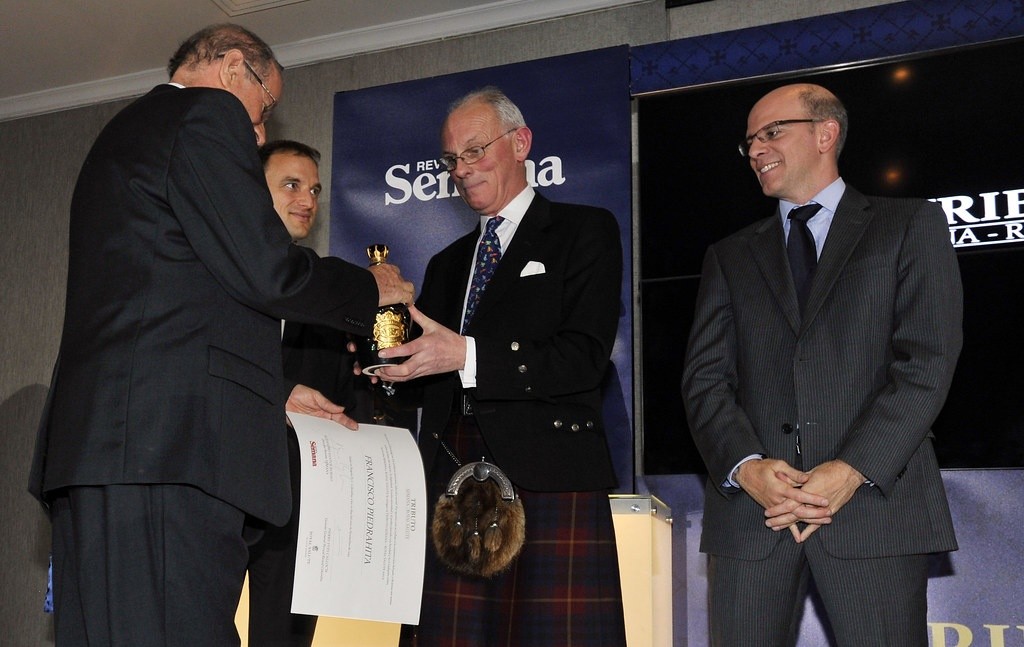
[349,86,629,647]
[679,82,965,647]
[27,21,417,647]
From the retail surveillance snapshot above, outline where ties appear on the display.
[460,216,505,336]
[786,204,823,318]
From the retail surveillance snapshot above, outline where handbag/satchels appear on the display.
[431,456,523,577]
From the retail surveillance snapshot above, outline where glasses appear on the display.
[739,118,825,156]
[217,54,276,123]
[438,128,516,173]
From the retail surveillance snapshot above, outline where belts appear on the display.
[452,394,473,417]
